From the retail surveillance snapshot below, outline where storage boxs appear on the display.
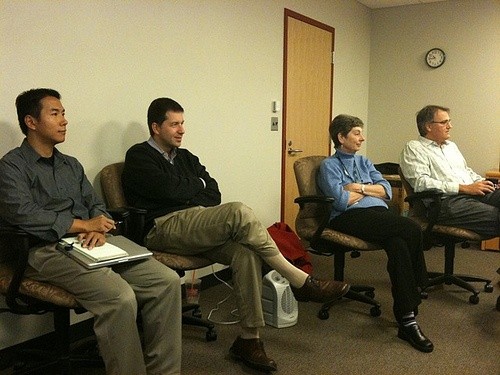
[382,175,409,211]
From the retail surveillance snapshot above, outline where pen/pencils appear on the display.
[114,221,123,225]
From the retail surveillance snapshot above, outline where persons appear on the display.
[123,97,352,372]
[398,105,500,312]
[0,88,183,375]
[317,114,435,353]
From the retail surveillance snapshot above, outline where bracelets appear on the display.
[361,184,366,193]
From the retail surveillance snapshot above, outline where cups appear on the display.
[184,279,201,305]
[486,177,498,191]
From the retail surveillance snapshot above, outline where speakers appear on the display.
[261,270,298,329]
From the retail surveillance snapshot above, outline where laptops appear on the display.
[57,235,154,270]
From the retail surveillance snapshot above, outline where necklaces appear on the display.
[335,152,364,184]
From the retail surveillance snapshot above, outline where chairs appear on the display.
[293,155,403,320]
[100,162,218,342]
[0,207,129,375]
[397,162,500,305]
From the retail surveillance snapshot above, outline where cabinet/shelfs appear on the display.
[480,170,499,252]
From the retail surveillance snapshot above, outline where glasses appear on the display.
[430,120,450,124]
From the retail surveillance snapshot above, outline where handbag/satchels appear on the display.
[266,221,313,275]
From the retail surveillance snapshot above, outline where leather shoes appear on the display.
[398,324,434,352]
[227,335,278,372]
[290,273,351,302]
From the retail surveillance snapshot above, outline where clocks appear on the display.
[425,47,445,68]
